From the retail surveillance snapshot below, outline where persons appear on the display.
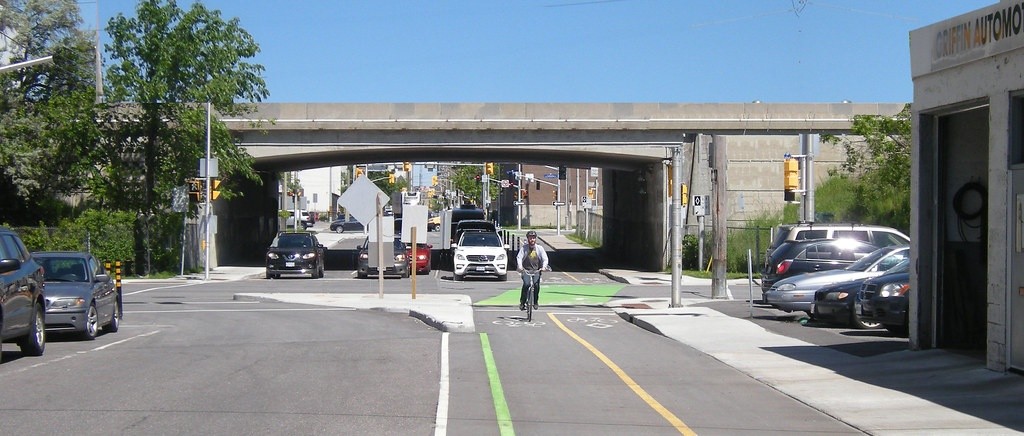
[517,231,548,311]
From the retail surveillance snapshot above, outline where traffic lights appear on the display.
[559,167,567,180]
[522,189,528,200]
[507,169,514,178]
[210,178,223,201]
[188,180,202,202]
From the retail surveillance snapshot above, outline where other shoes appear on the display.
[519,304,524,309]
[534,303,538,309]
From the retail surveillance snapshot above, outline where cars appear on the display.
[266,229,326,279]
[808,258,910,329]
[330,207,443,281]
[450,229,510,281]
[856,257,910,336]
[29,250,122,340]
[765,242,910,322]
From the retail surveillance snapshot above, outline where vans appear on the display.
[448,200,502,269]
[765,218,910,267]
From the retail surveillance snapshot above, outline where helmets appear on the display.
[526,231,537,236]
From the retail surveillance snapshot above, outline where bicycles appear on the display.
[521,267,552,322]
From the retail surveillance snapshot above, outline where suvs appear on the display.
[759,237,881,304]
[0,224,47,359]
[278,208,312,232]
